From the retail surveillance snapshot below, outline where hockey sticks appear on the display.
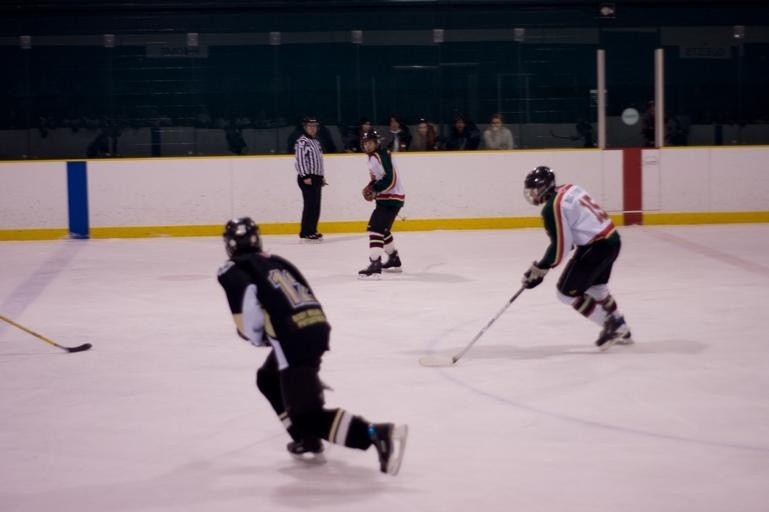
[0,315,92,352]
[418,286,526,367]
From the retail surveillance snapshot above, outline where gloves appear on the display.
[521,261,549,289]
[363,186,377,201]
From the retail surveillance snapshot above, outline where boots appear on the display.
[358,249,401,276]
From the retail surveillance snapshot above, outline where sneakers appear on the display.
[596,315,632,348]
[287,442,325,456]
[300,231,323,240]
[374,422,398,473]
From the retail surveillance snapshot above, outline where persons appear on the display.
[483,114,514,150]
[387,111,412,152]
[294,112,328,239]
[442,111,480,149]
[358,131,402,275]
[643,100,680,146]
[349,119,372,152]
[412,113,436,151]
[523,166,632,347]
[216,217,394,473]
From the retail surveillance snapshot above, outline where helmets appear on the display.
[222,218,261,261]
[360,128,382,154]
[521,166,556,205]
